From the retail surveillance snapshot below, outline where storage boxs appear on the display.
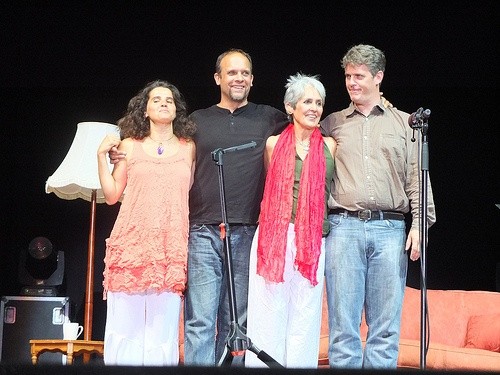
[0,296,78,375]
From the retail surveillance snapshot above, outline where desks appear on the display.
[30,340,104,365]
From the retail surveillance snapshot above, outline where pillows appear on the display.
[465,313,500,353]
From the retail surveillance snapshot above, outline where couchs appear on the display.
[179,287,500,373]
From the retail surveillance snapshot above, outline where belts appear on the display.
[329,208,405,220]
[204,223,255,226]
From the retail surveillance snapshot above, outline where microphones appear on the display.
[408,109,431,129]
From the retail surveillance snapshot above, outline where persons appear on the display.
[318,45,437,369]
[108,49,394,366]
[97,81,198,367]
[245,73,338,367]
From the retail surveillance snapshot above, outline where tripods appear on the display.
[211,141,284,369]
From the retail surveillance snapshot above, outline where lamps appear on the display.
[23,236,65,296]
[45,121,125,364]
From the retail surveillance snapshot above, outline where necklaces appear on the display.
[295,133,311,152]
[148,133,175,155]
